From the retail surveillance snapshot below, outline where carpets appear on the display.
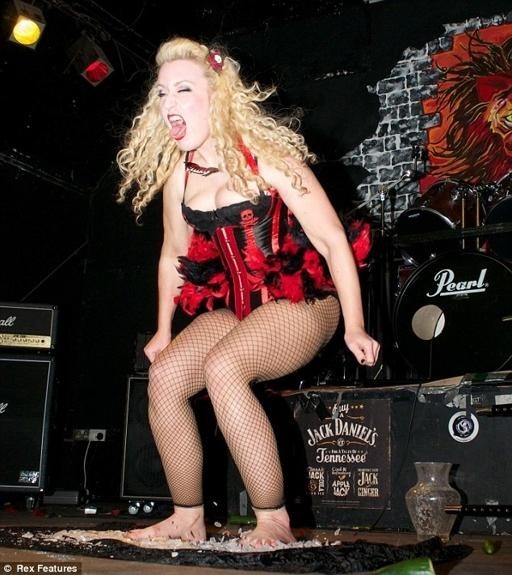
[0,521,476,574]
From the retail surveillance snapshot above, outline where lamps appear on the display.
[2,0,49,51]
[66,29,116,88]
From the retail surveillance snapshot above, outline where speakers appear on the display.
[214,390,391,531]
[0,354,56,493]
[391,394,512,537]
[119,370,213,505]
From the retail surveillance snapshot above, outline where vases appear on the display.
[404,461,464,544]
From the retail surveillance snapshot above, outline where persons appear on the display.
[116,37,381,551]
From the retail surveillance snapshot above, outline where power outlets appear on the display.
[89,428,107,444]
